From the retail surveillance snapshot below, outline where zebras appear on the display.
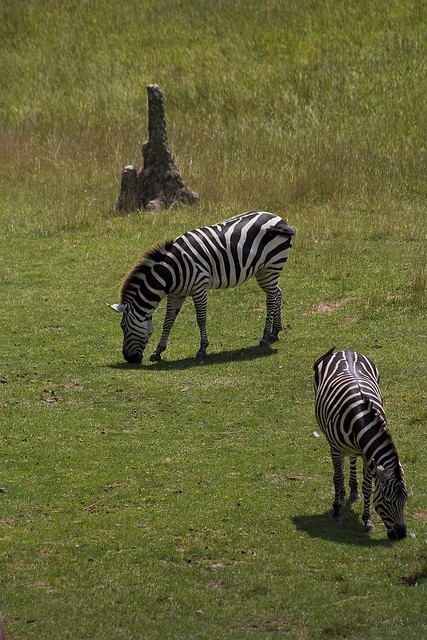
[312,347,409,540]
[105,210,296,364]
